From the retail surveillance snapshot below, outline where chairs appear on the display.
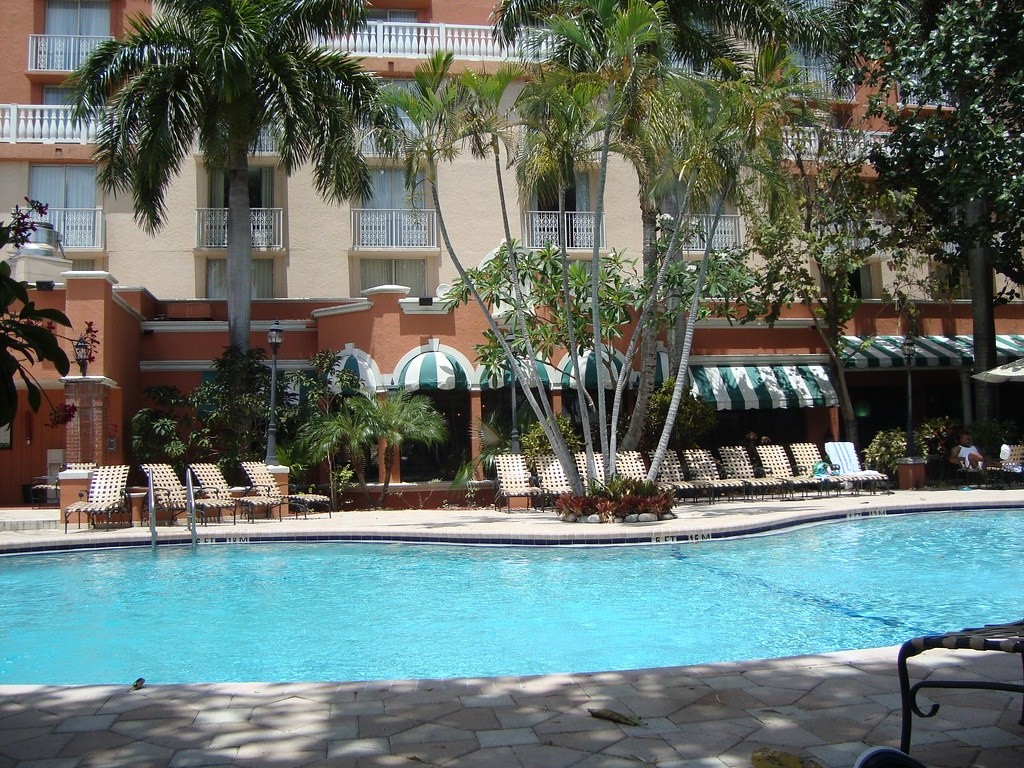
[825,441,897,495]
[683,450,745,504]
[494,453,544,511]
[140,464,237,528]
[893,619,1023,754]
[574,451,618,498]
[718,445,787,502]
[790,442,860,498]
[64,464,133,534]
[31,460,98,509]
[190,464,281,526]
[613,451,674,506]
[648,450,695,505]
[532,454,578,513]
[756,445,822,500]
[242,462,332,519]
[955,444,1024,490]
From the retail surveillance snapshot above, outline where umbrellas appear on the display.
[971,358,1024,383]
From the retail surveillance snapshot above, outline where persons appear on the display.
[950,434,1002,470]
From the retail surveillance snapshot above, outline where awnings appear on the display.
[687,360,839,410]
[561,350,627,389]
[843,333,1024,370]
[480,356,553,391]
[397,352,472,392]
[321,355,377,395]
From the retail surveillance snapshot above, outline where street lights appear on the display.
[265,319,284,465]
[902,335,916,456]
[505,333,520,453]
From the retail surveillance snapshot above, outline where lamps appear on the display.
[73,332,91,376]
